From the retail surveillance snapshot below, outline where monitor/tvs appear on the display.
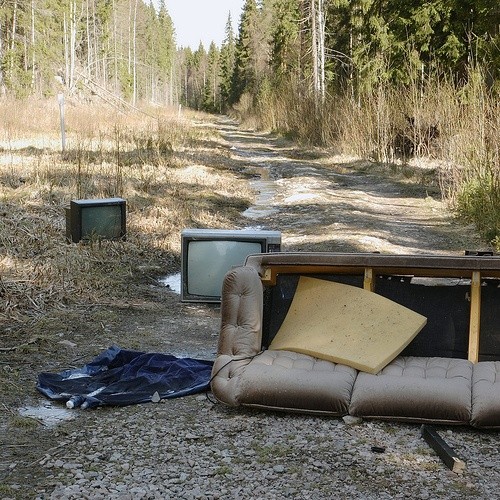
[181,229,281,303]
[65,196,126,247]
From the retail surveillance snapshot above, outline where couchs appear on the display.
[206,249,500,427]
